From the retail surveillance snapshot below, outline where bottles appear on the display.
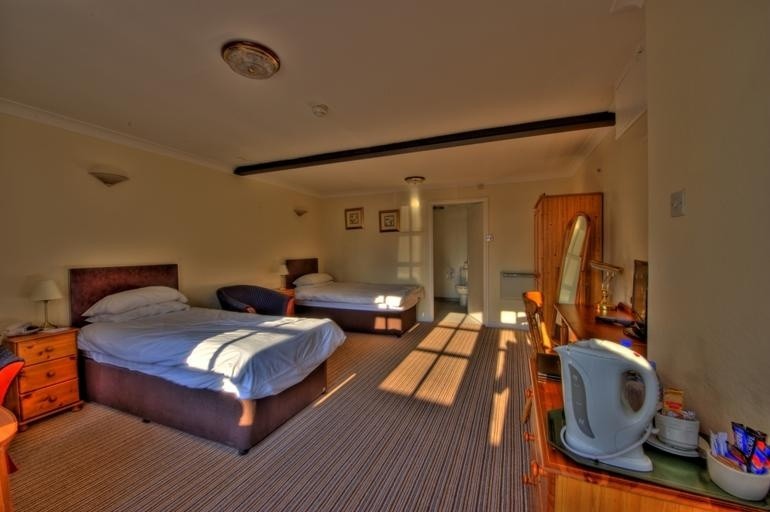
[646,360,662,428]
[620,339,638,381]
[662,388,686,415]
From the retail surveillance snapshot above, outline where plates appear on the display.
[641,432,710,461]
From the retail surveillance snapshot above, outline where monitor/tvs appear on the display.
[624,260,648,340]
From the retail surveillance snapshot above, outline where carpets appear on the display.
[8,320,536,512]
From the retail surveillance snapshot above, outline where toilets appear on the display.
[455,262,468,307]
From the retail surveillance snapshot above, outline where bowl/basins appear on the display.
[706,447,770,501]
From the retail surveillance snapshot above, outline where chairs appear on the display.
[0,346,27,475]
[521,288,561,424]
[214,282,297,316]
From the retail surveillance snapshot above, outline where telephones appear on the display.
[4,321,40,336]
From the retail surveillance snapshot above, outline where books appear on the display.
[535,352,562,382]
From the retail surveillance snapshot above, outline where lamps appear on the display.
[589,256,623,314]
[218,37,282,83]
[404,176,426,185]
[277,264,290,291]
[30,278,64,331]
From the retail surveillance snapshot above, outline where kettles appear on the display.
[549,339,661,476]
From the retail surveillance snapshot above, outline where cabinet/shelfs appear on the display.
[532,190,604,340]
[518,347,769,512]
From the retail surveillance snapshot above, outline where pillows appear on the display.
[293,270,334,284]
[83,300,191,322]
[78,283,189,316]
[297,282,334,288]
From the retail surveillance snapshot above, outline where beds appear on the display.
[67,262,348,458]
[281,257,426,338]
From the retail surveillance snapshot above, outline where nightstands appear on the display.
[272,287,296,317]
[3,327,86,434]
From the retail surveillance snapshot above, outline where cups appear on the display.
[656,411,704,451]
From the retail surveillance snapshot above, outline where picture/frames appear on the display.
[344,206,365,230]
[378,209,401,233]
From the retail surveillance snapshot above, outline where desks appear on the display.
[553,297,647,358]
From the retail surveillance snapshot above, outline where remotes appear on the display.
[596,317,635,327]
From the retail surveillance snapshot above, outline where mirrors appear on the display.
[552,210,593,331]
